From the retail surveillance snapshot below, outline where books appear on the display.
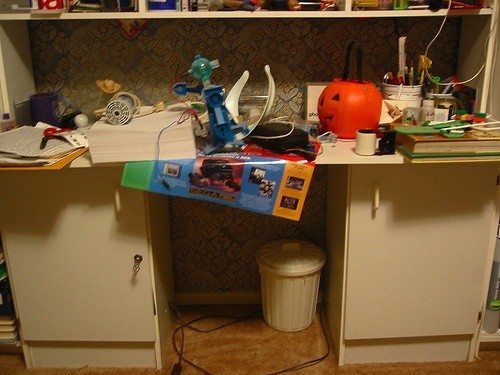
[389,122,500,159]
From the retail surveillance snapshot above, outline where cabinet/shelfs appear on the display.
[0,129,500,369]
[0,0,499,122]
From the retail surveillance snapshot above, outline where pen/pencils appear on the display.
[422,71,457,95]
[383,60,424,86]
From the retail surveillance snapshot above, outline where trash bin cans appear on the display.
[257,239,326,332]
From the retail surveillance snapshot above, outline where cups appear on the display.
[382,82,423,101]
[354,129,376,155]
[29,92,60,127]
[403,107,421,127]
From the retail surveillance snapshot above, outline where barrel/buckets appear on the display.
[255,239,327,332]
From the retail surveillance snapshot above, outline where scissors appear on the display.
[40,128,71,149]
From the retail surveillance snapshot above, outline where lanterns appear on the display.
[317,81,381,139]
